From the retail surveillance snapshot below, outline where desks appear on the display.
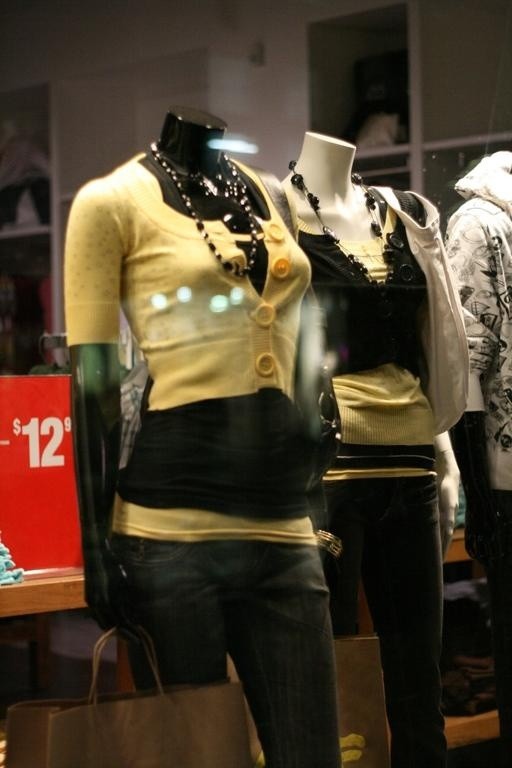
[342,523,503,766]
[1,562,139,698]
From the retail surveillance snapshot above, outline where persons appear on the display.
[280,130,469,768]
[64,103,344,768]
[444,150,511,766]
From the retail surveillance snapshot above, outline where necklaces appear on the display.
[150,140,259,276]
[288,160,395,303]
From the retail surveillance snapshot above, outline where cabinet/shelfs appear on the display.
[302,2,512,195]
[1,68,64,374]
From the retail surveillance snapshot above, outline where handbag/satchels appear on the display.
[4,621,255,768]
